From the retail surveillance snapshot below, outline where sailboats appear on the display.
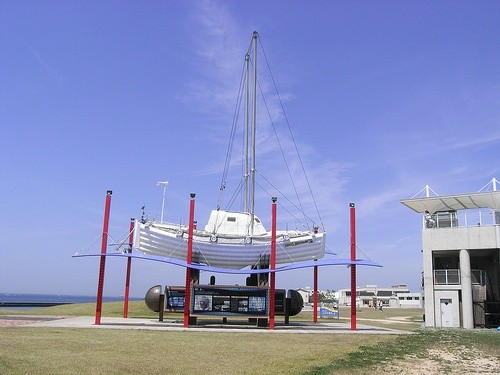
[130,29,327,269]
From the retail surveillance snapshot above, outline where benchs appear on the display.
[247,317,267,327]
